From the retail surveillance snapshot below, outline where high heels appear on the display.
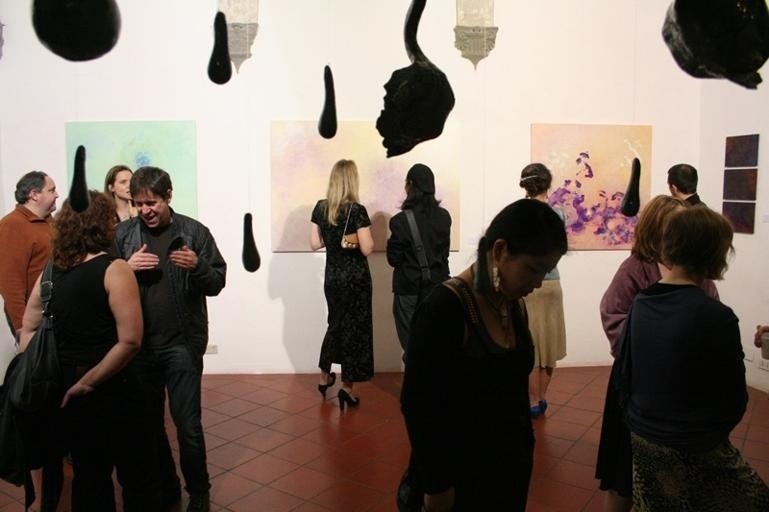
[318,372,336,398]
[338,388,360,411]
[530,400,547,419]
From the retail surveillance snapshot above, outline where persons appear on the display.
[397,197,568,511]
[308,158,375,411]
[594,193,721,510]
[111,166,226,512]
[0,171,60,347]
[664,163,703,206]
[20,188,162,510]
[754,324,768,348]
[102,165,139,230]
[518,162,566,418]
[613,205,751,511]
[386,164,452,381]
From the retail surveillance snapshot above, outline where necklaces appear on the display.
[470,262,511,348]
[117,210,130,221]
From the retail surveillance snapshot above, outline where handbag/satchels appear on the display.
[340,201,361,249]
[5,261,63,418]
[415,277,451,310]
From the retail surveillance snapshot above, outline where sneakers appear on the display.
[186,490,211,512]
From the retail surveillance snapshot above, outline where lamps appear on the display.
[218,2,259,71]
[453,0,499,69]
